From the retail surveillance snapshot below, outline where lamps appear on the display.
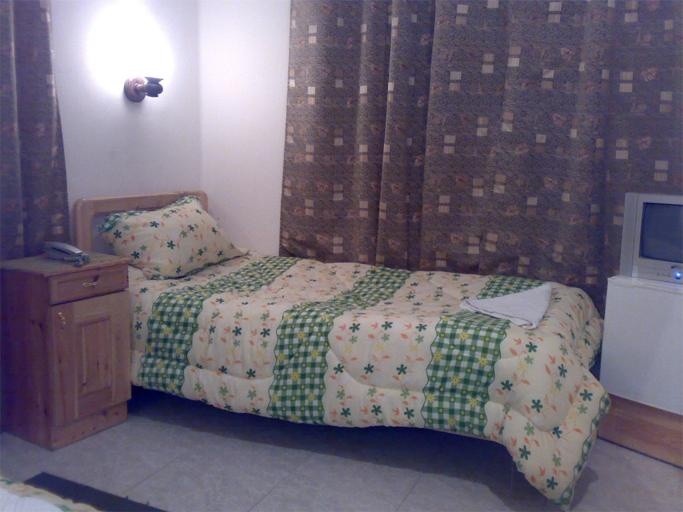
[124,75,163,102]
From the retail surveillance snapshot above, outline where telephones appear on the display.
[42,241,89,261]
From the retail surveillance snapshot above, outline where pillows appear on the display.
[97,193,250,281]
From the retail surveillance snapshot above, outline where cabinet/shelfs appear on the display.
[593,273,683,469]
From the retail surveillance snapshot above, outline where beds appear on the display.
[75,192,612,512]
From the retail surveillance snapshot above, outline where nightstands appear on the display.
[0,246,133,450]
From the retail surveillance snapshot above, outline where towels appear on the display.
[460,280,553,330]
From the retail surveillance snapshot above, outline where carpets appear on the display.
[23,470,163,511]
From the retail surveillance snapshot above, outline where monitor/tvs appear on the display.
[620,191,683,284]
